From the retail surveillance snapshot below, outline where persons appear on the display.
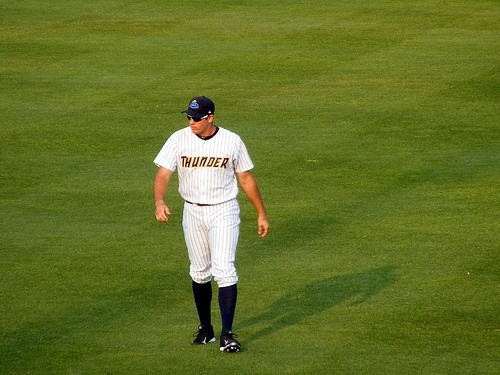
[153,96,270,352]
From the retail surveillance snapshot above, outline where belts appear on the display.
[185,200,213,206]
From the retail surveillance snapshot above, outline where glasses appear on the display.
[187,115,209,122]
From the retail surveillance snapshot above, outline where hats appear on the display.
[181,96,215,117]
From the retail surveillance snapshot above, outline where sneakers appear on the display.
[220,329,241,353]
[193,325,216,345]
